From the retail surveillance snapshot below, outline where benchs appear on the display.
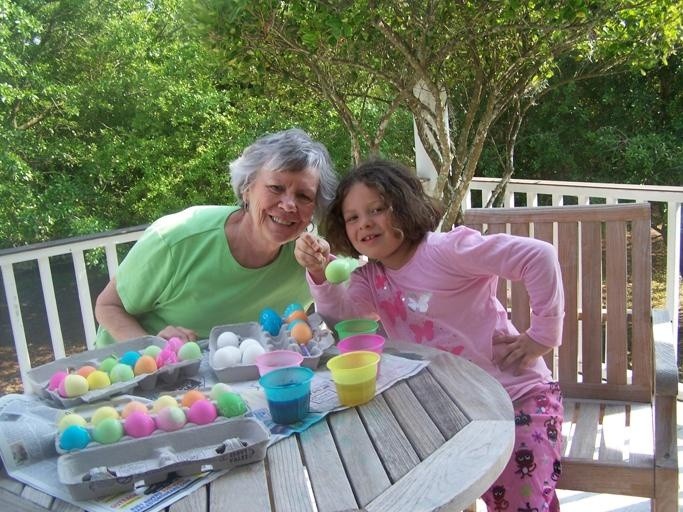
[455,203,679,512]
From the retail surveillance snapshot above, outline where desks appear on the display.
[0,338,516,511]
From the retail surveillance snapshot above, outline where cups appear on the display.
[325,351,380,405]
[337,334,386,380]
[255,350,303,377]
[335,318,378,341]
[259,367,314,423]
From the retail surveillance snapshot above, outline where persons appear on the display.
[94,127,341,351]
[293,157,567,512]
